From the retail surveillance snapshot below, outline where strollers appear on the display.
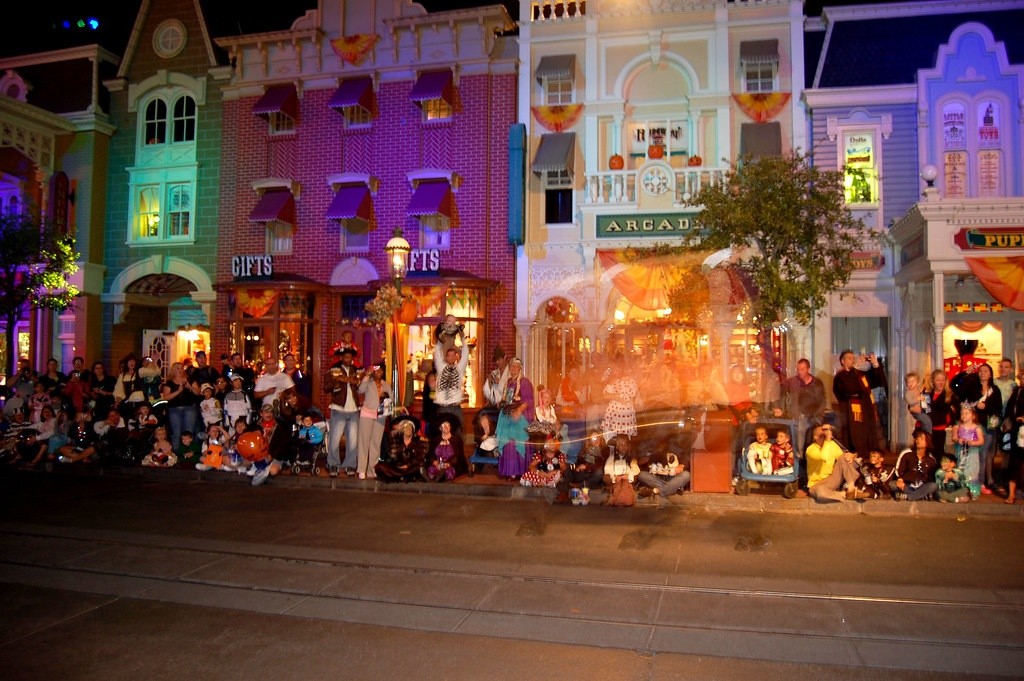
[113,402,161,466]
[734,419,801,499]
[291,407,327,476]
[468,405,500,472]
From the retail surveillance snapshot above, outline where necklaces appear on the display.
[374,381,383,399]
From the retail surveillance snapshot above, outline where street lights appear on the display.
[384,225,411,417]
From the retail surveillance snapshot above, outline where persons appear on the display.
[0,324,1024,505]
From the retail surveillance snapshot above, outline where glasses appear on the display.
[215,379,226,384]
[74,361,83,365]
[263,408,273,414]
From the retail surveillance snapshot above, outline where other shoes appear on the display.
[194,463,208,472]
[329,465,340,477]
[508,474,516,482]
[344,467,355,477]
[895,493,907,502]
[358,472,365,479]
[640,486,654,498]
[419,466,431,483]
[846,487,870,500]
[922,492,933,502]
[434,468,446,483]
[677,486,684,495]
[59,456,72,463]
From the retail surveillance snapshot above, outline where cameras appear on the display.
[865,356,871,361]
[373,365,381,370]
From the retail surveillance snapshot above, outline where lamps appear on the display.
[954,278,966,290]
[919,163,940,187]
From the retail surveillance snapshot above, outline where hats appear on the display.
[502,357,523,400]
[229,373,244,382]
[200,383,213,394]
[390,415,420,434]
[428,411,460,432]
[334,347,358,356]
